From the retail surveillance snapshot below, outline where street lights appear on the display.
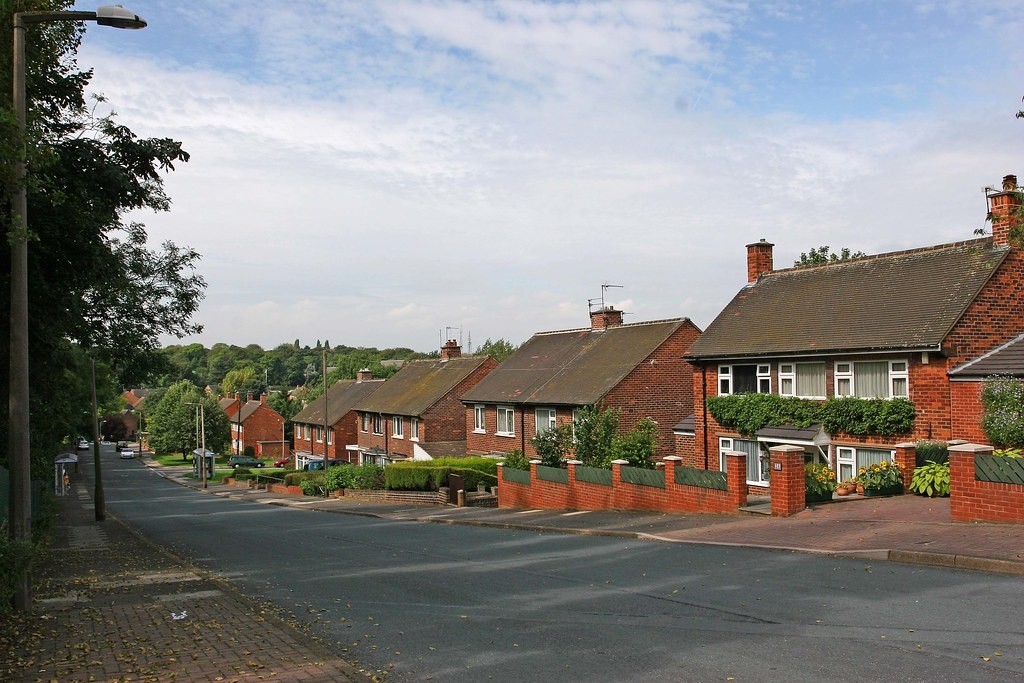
[277,418,284,460]
[99,421,107,441]
[9,8,149,611]
[185,402,207,488]
[130,410,143,457]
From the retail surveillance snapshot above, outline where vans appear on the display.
[304,459,352,471]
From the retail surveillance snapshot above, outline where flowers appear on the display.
[834,478,864,489]
[859,461,906,489]
[804,461,837,495]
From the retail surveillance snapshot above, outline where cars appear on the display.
[274,456,294,468]
[101,440,111,446]
[120,448,135,459]
[78,436,89,450]
[226,456,265,469]
[115,440,128,452]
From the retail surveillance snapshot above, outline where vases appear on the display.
[836,487,848,496]
[865,484,904,496]
[843,482,856,493]
[856,484,865,495]
[806,491,832,503]
